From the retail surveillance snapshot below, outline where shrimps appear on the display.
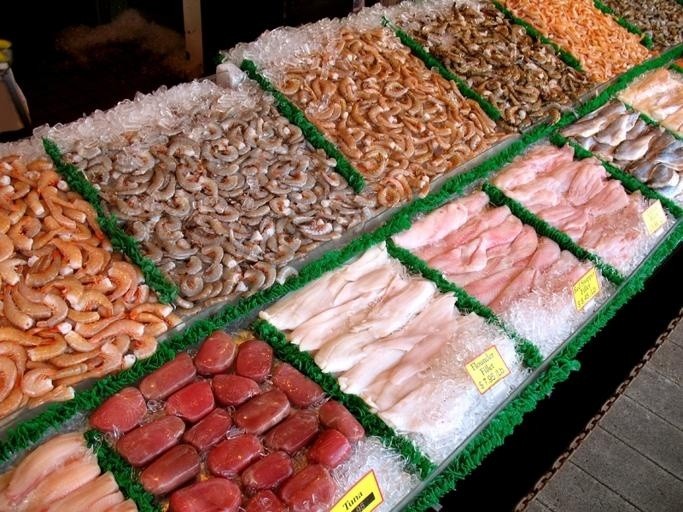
[0,0,683,417]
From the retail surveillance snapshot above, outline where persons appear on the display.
[53,0,213,109]
